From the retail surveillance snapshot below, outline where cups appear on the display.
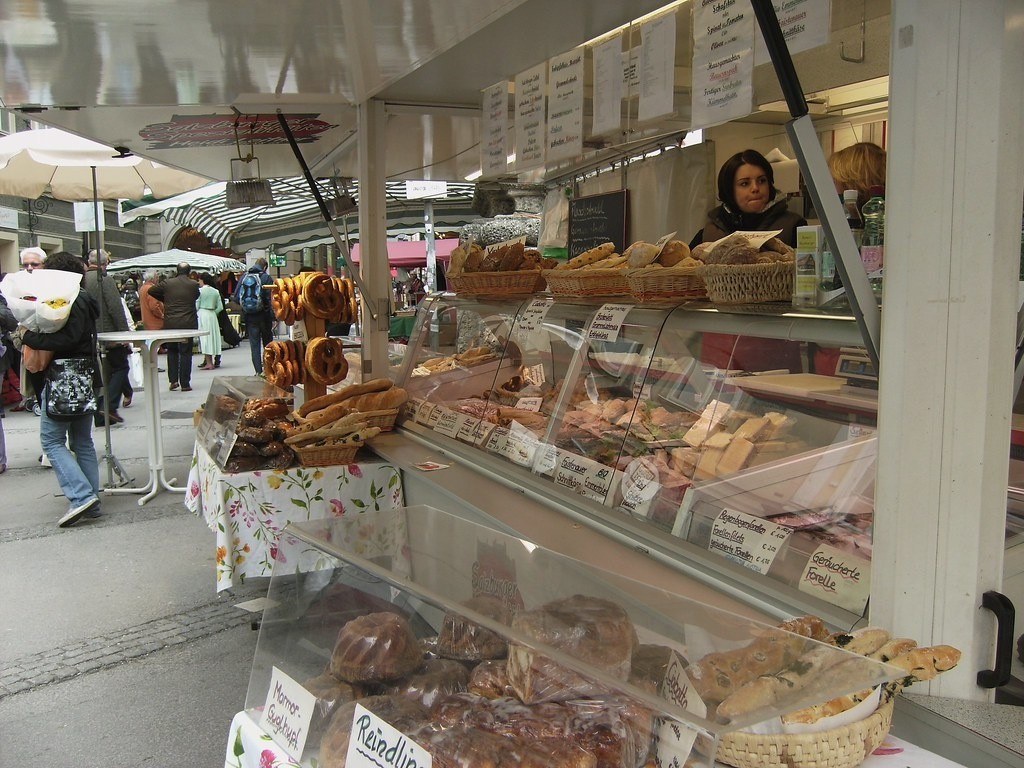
[393,343,407,354]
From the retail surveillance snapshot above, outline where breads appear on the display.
[684,617,961,726]
[672,398,809,482]
[461,241,543,271]
[550,236,798,271]
[210,272,408,473]
[423,347,489,373]
[301,603,692,768]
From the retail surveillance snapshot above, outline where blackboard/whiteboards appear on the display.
[568,190,627,255]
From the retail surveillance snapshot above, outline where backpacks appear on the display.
[237,272,270,315]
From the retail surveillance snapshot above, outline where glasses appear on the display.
[22,262,42,268]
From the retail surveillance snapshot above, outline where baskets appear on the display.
[699,260,795,305]
[620,266,709,302]
[445,270,547,295]
[693,696,894,768]
[283,408,400,466]
[541,269,630,297]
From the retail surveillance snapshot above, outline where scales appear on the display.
[834,341,880,387]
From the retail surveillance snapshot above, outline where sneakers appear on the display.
[82,507,100,518]
[57,498,99,527]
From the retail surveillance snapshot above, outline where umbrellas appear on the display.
[1,129,217,495]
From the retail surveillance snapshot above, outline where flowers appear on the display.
[0,269,84,374]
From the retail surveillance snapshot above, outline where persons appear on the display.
[195,272,223,370]
[0,246,134,528]
[688,148,807,373]
[407,270,426,306]
[139,267,166,372]
[190,271,238,300]
[233,257,279,376]
[118,270,141,325]
[148,262,200,391]
[810,142,887,376]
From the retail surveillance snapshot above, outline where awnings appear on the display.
[121,177,477,256]
[349,238,459,265]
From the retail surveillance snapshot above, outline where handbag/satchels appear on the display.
[46,358,100,421]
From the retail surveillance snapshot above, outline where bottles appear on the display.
[861,185,885,290]
[821,190,863,309]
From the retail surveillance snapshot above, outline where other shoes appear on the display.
[123,398,131,407]
[200,365,215,370]
[214,361,221,366]
[0,464,6,473]
[182,387,192,391]
[9,405,26,412]
[95,421,117,427]
[169,382,179,390]
[197,360,207,367]
[158,368,165,372]
[99,409,123,422]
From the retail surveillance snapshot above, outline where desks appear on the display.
[92,329,211,505]
[183,440,411,630]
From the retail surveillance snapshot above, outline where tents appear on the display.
[107,248,245,272]
